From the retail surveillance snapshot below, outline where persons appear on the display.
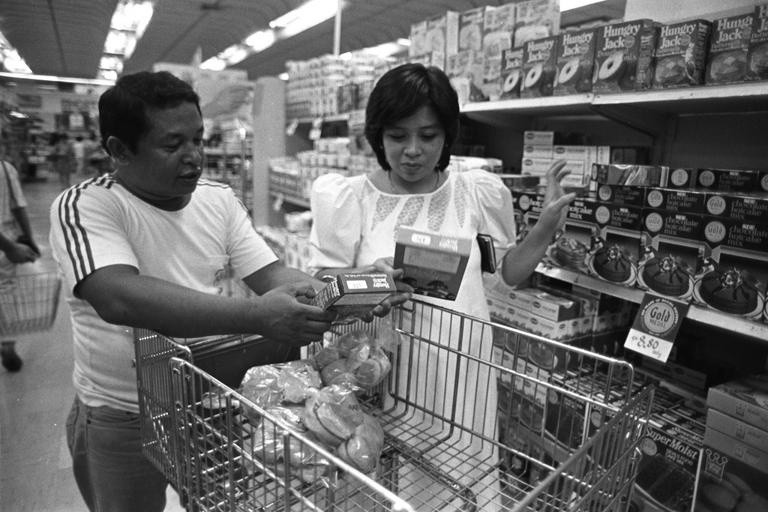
[309,62,577,512]
[49,70,411,512]
[0,132,108,371]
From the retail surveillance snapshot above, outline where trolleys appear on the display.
[0,251,61,335]
[132,293,656,512]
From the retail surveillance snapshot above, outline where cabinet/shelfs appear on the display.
[266,84,768,511]
